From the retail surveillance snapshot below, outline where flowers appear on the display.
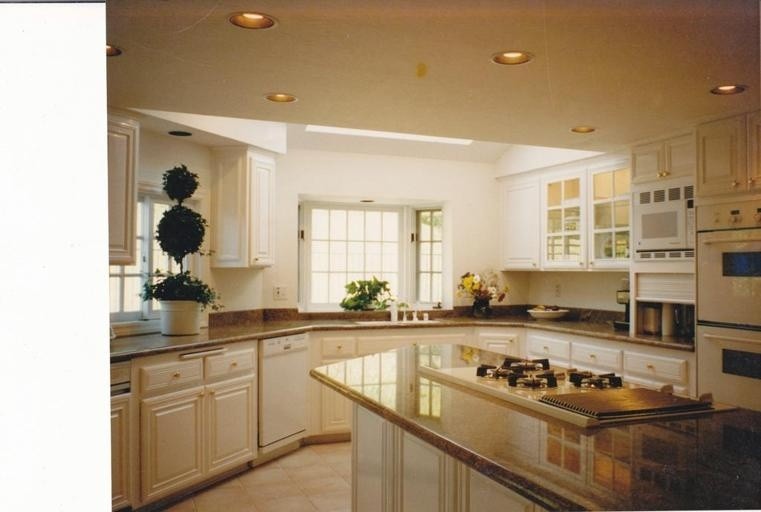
[457,263,513,306]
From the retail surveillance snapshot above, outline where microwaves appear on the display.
[635,199,695,254]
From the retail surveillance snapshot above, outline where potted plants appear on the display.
[339,272,413,313]
[138,159,227,337]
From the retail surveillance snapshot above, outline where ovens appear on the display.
[695,194,761,413]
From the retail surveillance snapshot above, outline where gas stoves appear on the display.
[418,356,738,432]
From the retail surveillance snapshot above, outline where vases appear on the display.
[472,298,494,320]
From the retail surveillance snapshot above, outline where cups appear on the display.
[621,278,628,289]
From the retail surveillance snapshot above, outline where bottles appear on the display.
[675,304,691,336]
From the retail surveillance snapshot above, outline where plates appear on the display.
[527,310,569,320]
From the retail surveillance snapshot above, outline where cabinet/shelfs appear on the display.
[350,402,399,511]
[313,327,356,449]
[400,427,456,511]
[499,170,544,271]
[625,340,698,402]
[212,144,280,270]
[479,325,527,363]
[108,105,144,266]
[572,333,625,385]
[540,147,636,271]
[356,327,480,360]
[527,327,572,369]
[140,338,259,511]
[111,354,139,512]
[456,458,536,511]
[637,131,698,184]
[692,109,761,201]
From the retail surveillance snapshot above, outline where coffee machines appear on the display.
[613,291,629,329]
